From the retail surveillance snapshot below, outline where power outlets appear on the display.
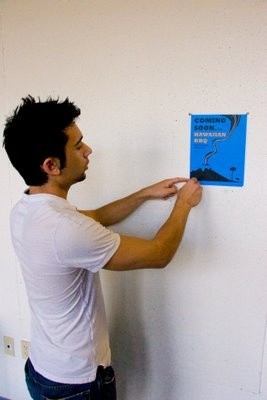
[21,340,30,360]
[4,336,15,357]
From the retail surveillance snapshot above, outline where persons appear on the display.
[2,94,204,400]
